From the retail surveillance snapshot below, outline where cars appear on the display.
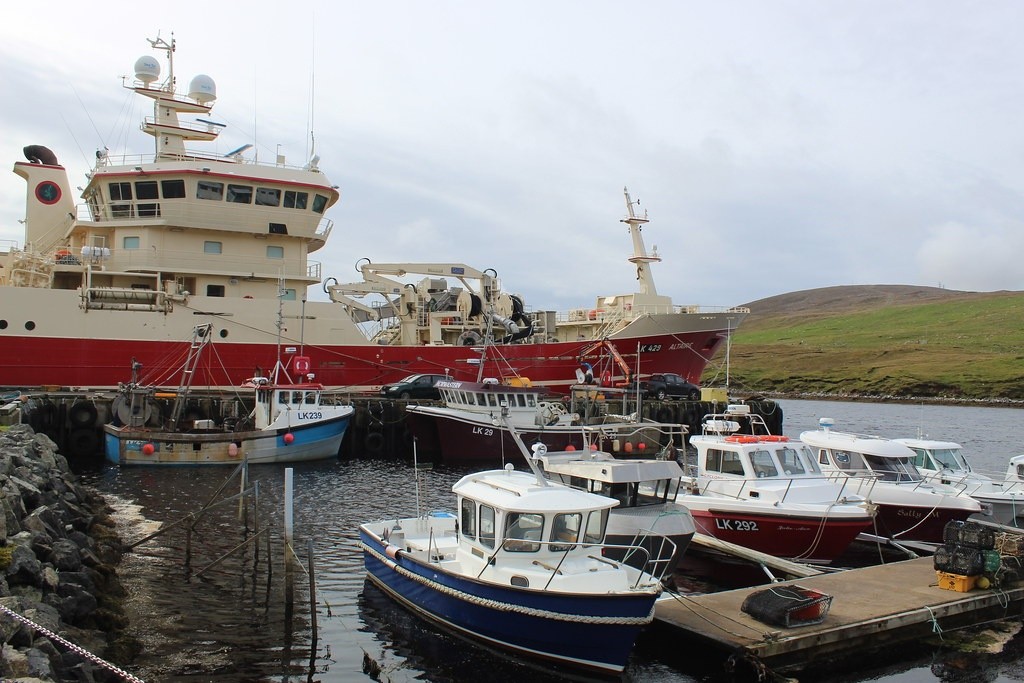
[641,372,702,403]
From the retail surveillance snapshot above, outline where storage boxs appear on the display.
[937,571,979,592]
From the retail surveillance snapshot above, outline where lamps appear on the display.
[96,150,106,158]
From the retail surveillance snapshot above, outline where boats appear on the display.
[631,403,879,592]
[405,311,666,466]
[1002,453,1024,490]
[100,321,354,468]
[886,400,1024,528]
[0,29,752,446]
[527,425,697,582]
[355,401,665,683]
[754,413,983,554]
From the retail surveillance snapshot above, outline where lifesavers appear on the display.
[57,249,72,259]
[724,434,758,444]
[755,435,789,443]
